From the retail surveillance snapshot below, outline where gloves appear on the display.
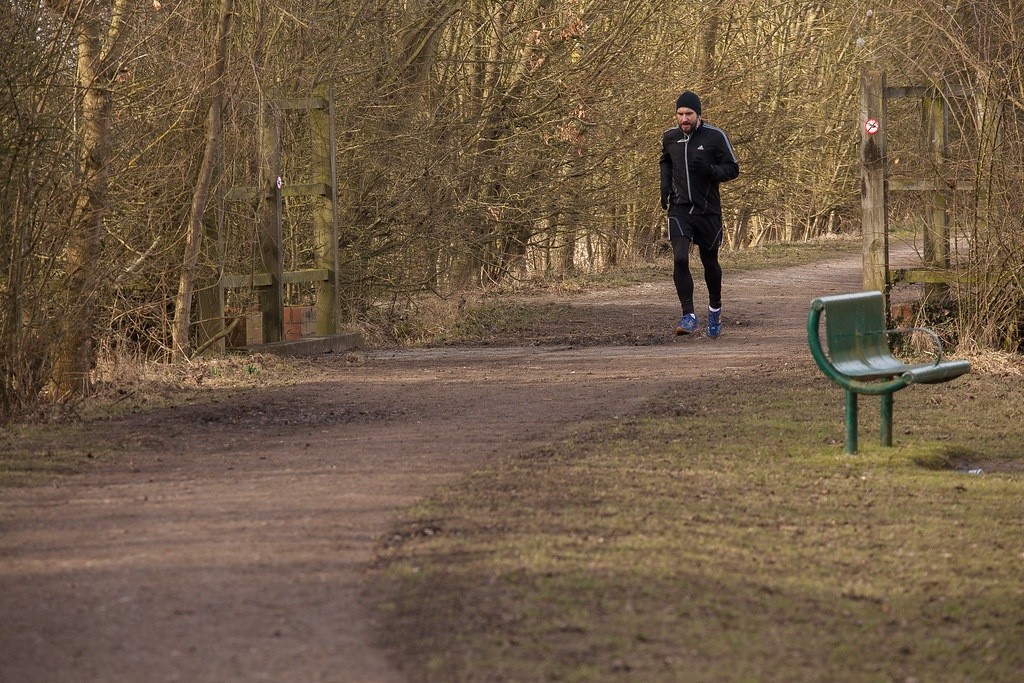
[661,186,674,210]
[689,157,713,177]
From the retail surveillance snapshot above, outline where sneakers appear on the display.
[676,313,697,333]
[706,303,722,337]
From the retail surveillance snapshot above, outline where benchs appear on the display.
[805,292,972,455]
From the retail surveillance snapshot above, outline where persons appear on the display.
[660,92,739,337]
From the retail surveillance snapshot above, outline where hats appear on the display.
[676,91,701,115]
